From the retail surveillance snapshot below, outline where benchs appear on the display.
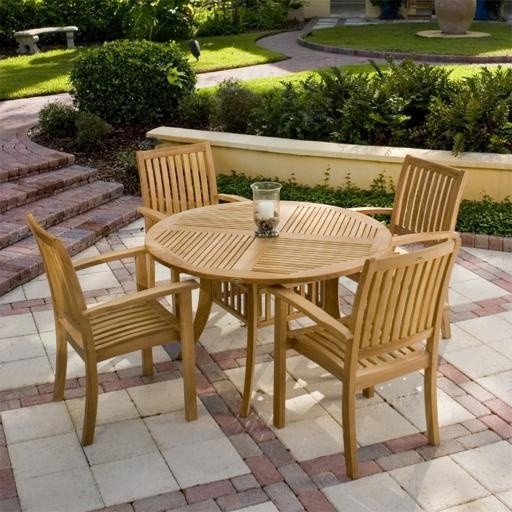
[13,26,79,54]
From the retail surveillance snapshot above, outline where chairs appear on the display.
[134,141,266,318]
[25,213,204,447]
[266,230,462,482]
[320,153,469,340]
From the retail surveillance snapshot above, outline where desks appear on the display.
[142,199,393,429]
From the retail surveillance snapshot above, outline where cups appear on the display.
[251,182,283,238]
